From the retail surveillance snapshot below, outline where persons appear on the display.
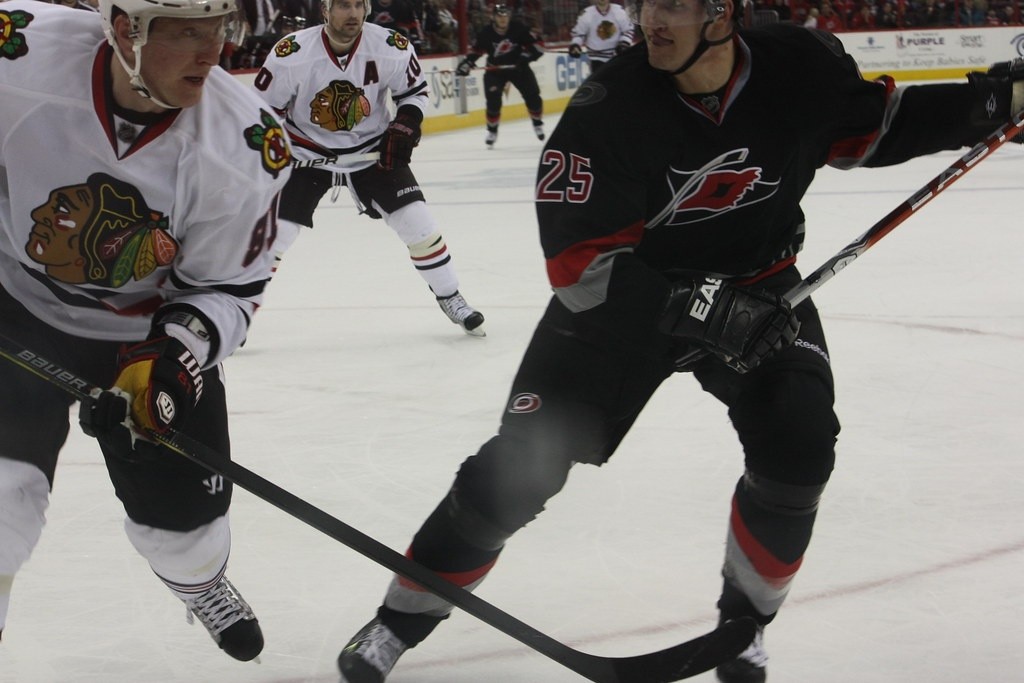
[250,0,487,340]
[0,0,295,670]
[47,1,1024,73]
[337,0,1023,683]
[455,0,547,149]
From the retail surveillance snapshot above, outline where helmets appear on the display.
[321,1,371,16]
[101,0,237,47]
[493,4,510,17]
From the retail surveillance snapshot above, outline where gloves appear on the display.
[615,42,630,55]
[656,276,801,373]
[379,105,425,174]
[967,61,1024,142]
[569,43,583,58]
[514,51,534,70]
[79,309,221,449]
[455,54,481,76]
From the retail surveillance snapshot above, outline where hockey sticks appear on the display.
[440,64,517,74]
[0,332,758,683]
[292,152,381,169]
[656,107,1024,373]
[544,50,618,56]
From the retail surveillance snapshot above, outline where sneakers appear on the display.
[435,289,486,338]
[484,133,498,150]
[712,596,774,683]
[534,123,546,141]
[171,578,266,665]
[337,614,408,683]
